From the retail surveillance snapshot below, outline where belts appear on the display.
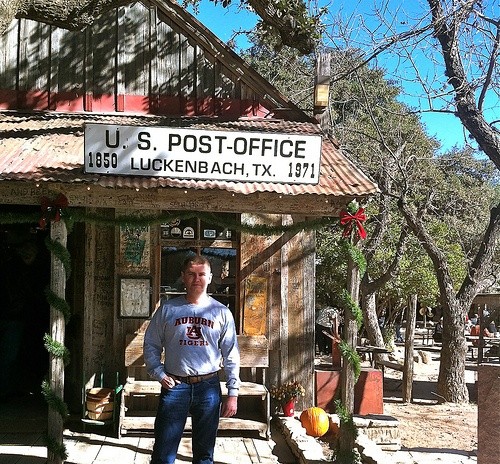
[165,372,218,384]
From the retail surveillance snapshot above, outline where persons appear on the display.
[143,256,241,464]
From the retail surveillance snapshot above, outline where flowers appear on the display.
[270,379,306,409]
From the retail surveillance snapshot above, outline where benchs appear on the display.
[117,332,274,440]
[413,326,500,365]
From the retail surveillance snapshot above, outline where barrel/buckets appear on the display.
[86,388,115,421]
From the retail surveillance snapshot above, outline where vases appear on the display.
[279,399,296,418]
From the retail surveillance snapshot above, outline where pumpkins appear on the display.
[300,405,330,437]
